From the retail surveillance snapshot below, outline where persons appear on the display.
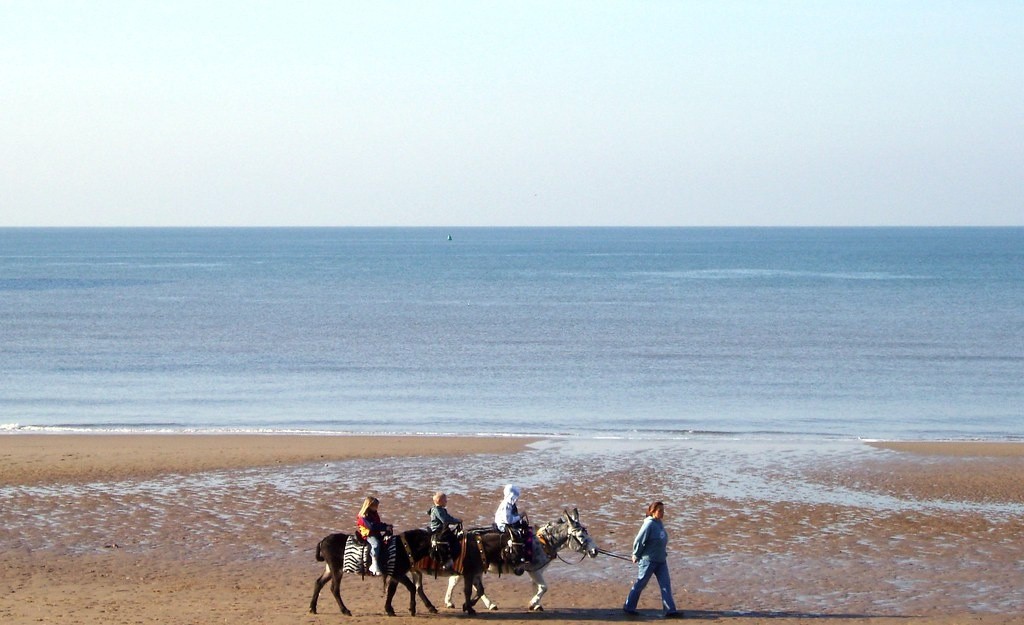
[426,491,463,573]
[622,500,686,618]
[356,495,394,577]
[493,482,533,571]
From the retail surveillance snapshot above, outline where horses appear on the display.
[309,526,450,618]
[445,506,598,610]
[381,514,529,610]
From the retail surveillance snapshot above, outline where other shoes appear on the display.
[664,612,683,618]
[623,607,640,616]
